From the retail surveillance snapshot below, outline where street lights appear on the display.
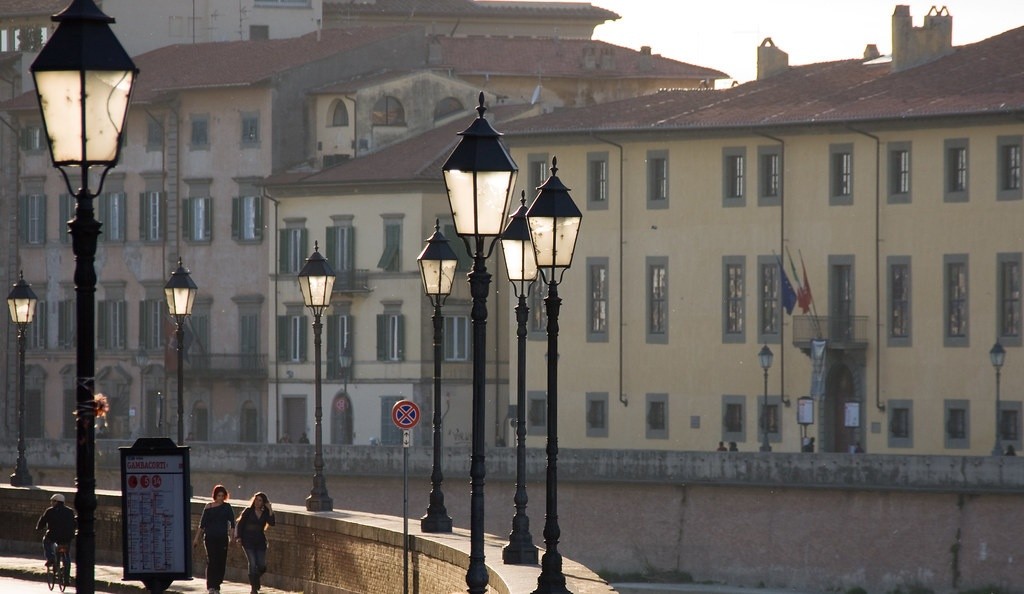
[989,337,1005,453]
[757,344,777,452]
[165,257,201,448]
[157,391,165,432]
[8,268,38,485]
[417,217,459,533]
[440,86,523,594]
[30,0,145,594]
[521,153,585,594]
[496,189,540,567]
[296,239,337,513]
[340,346,357,448]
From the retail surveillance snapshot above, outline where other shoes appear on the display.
[208,588,220,594]
[45,559,54,566]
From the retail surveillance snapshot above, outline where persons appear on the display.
[234,492,276,594]
[96,426,107,439]
[855,442,864,453]
[1006,445,1016,456]
[717,441,727,451]
[728,441,738,451]
[193,484,235,594]
[185,432,195,441]
[299,433,309,443]
[496,435,506,447]
[805,437,815,452]
[279,433,292,444]
[36,493,76,586]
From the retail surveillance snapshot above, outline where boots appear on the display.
[248,570,263,594]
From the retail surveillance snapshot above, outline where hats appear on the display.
[50,493,65,503]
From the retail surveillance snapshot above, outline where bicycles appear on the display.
[35,528,70,592]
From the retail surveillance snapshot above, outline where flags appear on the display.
[163,319,193,372]
[779,263,812,315]
